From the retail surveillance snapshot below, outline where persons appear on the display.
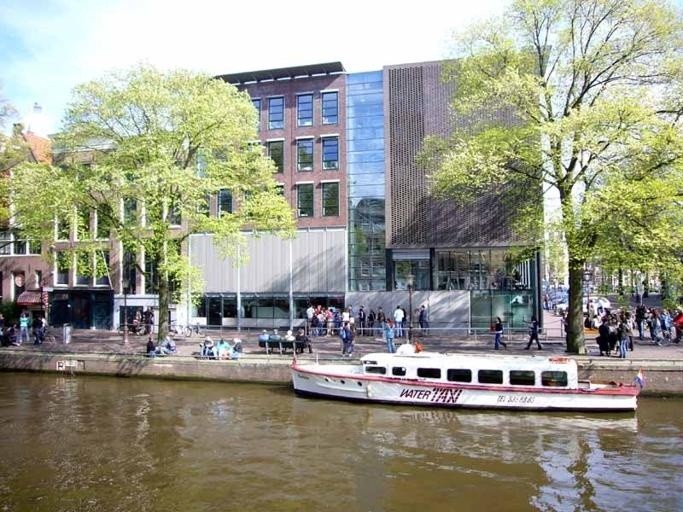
[560,311,568,352]
[259,302,430,357]
[494,317,506,350]
[132,306,154,335]
[147,336,176,356]
[0,311,49,345]
[584,298,683,358]
[199,335,242,360]
[544,293,551,310]
[523,315,542,350]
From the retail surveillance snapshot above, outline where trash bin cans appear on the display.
[63,322,71,345]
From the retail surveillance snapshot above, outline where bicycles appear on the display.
[169,319,206,337]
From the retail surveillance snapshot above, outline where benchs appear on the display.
[200,340,234,359]
[259,340,307,355]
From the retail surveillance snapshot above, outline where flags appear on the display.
[635,365,646,388]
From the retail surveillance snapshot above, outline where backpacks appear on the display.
[339,330,346,339]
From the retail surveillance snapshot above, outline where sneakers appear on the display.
[263,348,313,353]
[523,345,542,349]
[638,335,682,343]
[342,353,352,358]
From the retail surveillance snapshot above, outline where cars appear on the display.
[544,287,610,315]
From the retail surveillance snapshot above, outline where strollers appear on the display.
[661,325,677,347]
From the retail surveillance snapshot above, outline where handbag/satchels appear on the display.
[345,343,354,352]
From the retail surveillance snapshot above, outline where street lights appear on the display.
[582,271,593,329]
[406,274,415,329]
[40,280,45,326]
[121,278,130,343]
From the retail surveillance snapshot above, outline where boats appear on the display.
[288,344,640,411]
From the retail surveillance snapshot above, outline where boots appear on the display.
[494,342,507,349]
